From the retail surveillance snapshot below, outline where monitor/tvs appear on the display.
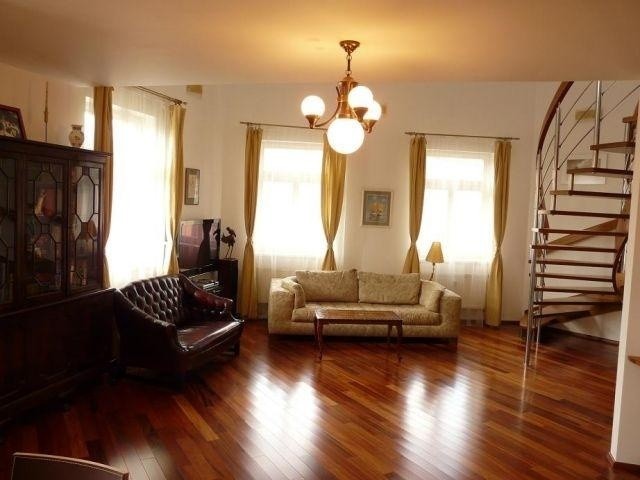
[176,219,221,267]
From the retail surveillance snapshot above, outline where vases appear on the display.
[69,125,84,148]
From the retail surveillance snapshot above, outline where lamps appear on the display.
[300,39,382,155]
[425,241,445,281]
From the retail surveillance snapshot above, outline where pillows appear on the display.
[295,270,358,302]
[358,271,421,304]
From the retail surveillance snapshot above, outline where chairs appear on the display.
[9,452,130,480]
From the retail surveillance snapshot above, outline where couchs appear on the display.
[267,269,462,352]
[114,271,245,392]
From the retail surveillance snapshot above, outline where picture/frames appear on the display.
[185,167,200,205]
[560,151,607,185]
[0,104,27,140]
[360,189,393,228]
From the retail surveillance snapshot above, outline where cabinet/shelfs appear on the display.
[178,258,238,317]
[0,137,117,428]
